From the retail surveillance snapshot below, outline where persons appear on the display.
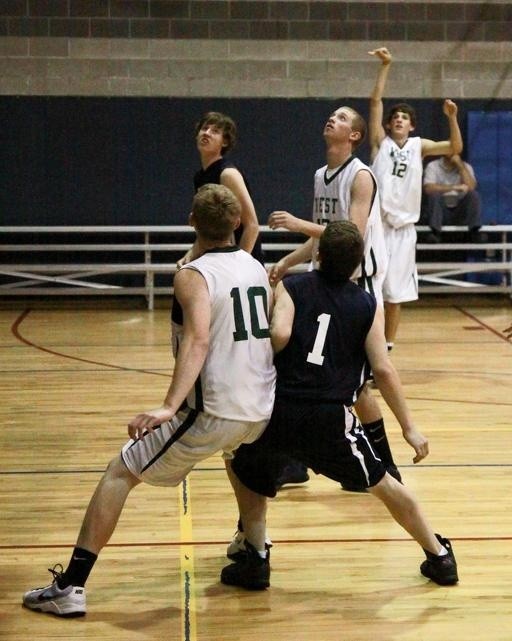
[425,152,482,240]
[369,46,464,352]
[177,109,266,274]
[225,221,459,587]
[465,221,504,297]
[20,186,277,617]
[269,105,384,312]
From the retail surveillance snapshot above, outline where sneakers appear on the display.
[221,524,272,591]
[420,533,458,586]
[23,564,87,617]
